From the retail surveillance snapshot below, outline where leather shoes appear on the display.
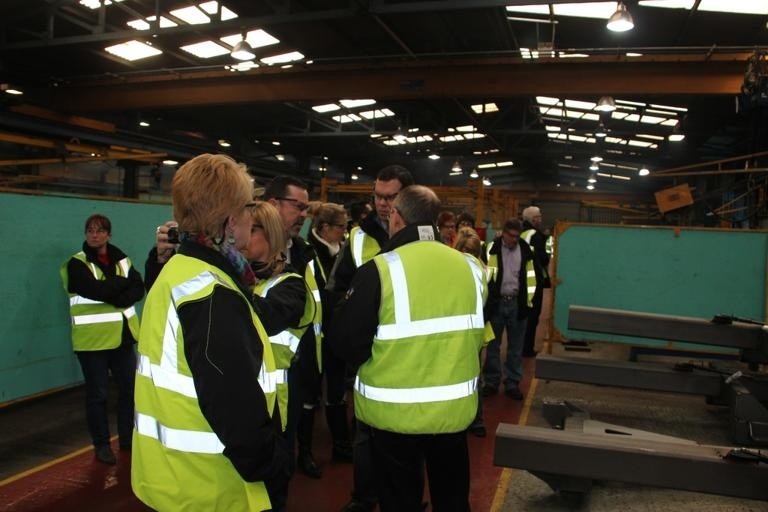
[471,427,487,438]
[481,385,498,397]
[504,388,524,401]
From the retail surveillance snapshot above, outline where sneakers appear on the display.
[95,444,115,466]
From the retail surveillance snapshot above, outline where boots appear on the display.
[325,405,353,463]
[296,406,323,477]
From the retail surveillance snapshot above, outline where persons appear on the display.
[146,164,413,478]
[126,151,289,512]
[58,213,146,492]
[328,186,487,509]
[439,206,554,438]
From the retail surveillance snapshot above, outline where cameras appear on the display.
[164,226,183,245]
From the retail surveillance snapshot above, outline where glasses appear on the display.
[274,196,309,210]
[325,222,348,230]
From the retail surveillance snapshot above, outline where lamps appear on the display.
[448,160,466,178]
[586,95,615,190]
[605,0,637,32]
[482,175,497,187]
[469,169,480,178]
[232,28,256,62]
